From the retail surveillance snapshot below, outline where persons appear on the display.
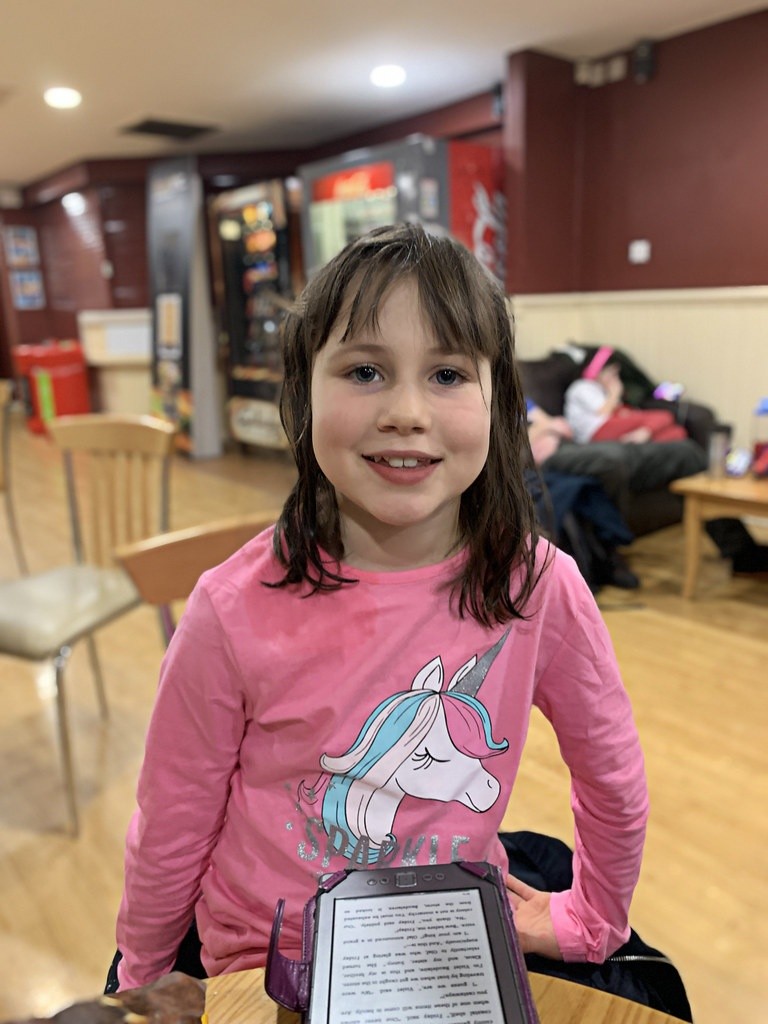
[565,346,688,443]
[117,222,648,993]
[525,397,640,589]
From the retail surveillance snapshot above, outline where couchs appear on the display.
[518,344,718,541]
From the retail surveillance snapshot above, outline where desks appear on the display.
[668,471,768,601]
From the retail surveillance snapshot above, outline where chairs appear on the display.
[115,504,281,650]
[1,412,181,837]
